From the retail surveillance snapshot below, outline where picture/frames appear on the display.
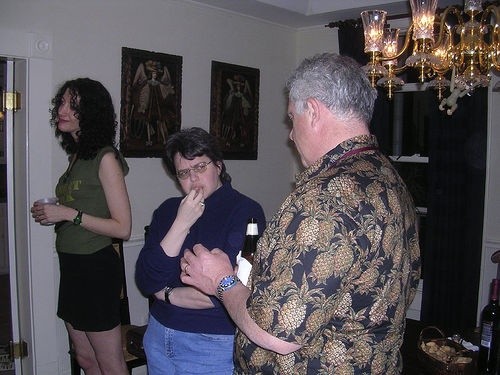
[208,58,260,162]
[118,44,183,162]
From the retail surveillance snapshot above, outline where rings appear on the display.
[185,264,190,274]
[200,201,204,205]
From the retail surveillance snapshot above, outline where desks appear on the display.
[416,325,500,375]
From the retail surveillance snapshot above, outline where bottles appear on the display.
[237,217,259,287]
[478,279,500,375]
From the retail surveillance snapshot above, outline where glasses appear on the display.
[176,160,212,181]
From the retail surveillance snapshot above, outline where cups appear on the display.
[34,197,59,225]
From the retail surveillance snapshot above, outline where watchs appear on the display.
[165,286,175,304]
[217,275,240,303]
[73,208,83,225]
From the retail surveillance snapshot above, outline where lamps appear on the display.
[361,0,500,103]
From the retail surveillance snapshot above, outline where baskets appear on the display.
[417,326,474,375]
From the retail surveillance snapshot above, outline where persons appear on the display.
[31,78,131,375]
[179,53,421,375]
[134,127,265,375]
